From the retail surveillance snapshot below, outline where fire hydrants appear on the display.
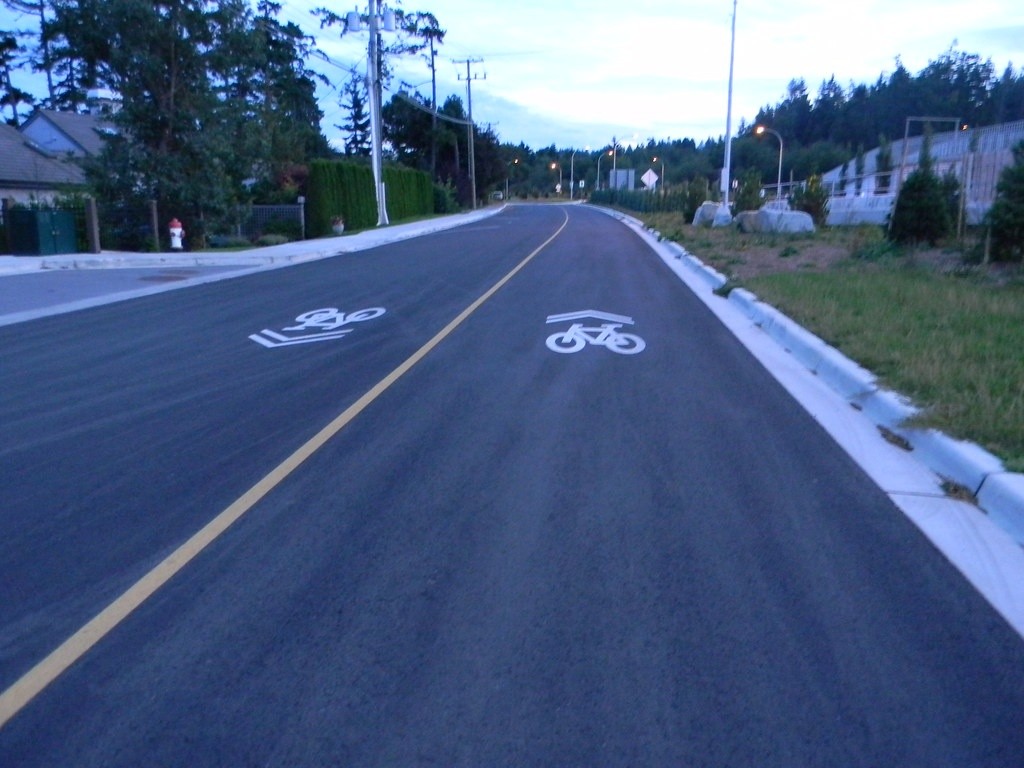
[169,217,186,252]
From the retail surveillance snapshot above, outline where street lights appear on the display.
[597,150,613,190]
[504,158,518,199]
[757,126,782,199]
[653,157,664,190]
[569,146,590,201]
[551,162,562,188]
